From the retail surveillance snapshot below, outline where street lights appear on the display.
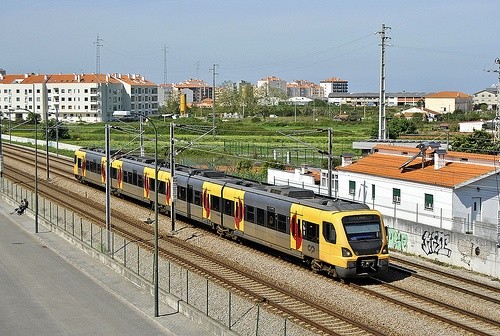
[7,106,37,234]
[112,110,160,317]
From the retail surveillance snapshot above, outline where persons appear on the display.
[9,199,24,215]
[17,198,29,215]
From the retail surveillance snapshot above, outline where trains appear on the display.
[72,146,392,284]
